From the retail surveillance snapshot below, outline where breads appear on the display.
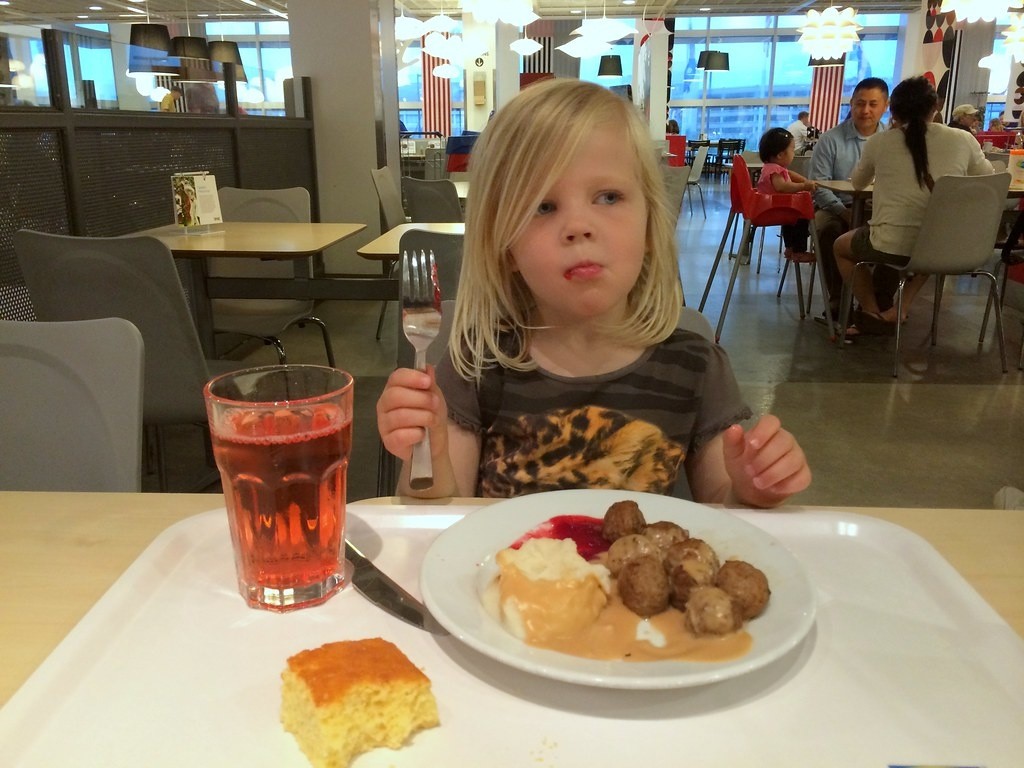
[281,637,441,768]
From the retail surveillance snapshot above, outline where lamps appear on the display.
[696,51,730,71]
[128,0,248,83]
[796,0,864,68]
[394,0,640,79]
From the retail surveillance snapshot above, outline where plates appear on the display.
[982,147,1010,153]
[418,486,815,690]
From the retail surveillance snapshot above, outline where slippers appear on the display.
[840,311,897,342]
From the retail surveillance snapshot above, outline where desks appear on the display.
[684,139,740,179]
[356,222,464,340]
[452,179,470,200]
[723,163,763,185]
[814,130,1024,336]
[1,491,1024,703]
[115,222,367,494]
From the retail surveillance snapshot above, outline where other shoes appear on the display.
[791,250,816,263]
[784,248,794,258]
[813,308,839,338]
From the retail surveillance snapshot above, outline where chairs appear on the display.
[838,172,1012,375]
[370,167,696,499]
[664,138,815,343]
[1,186,334,493]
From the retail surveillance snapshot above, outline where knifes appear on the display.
[342,540,450,637]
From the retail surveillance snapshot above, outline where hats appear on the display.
[951,104,980,117]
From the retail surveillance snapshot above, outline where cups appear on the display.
[984,142,992,151]
[203,361,355,612]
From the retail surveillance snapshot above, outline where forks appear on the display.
[399,248,443,491]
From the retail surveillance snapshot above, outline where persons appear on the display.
[811,77,900,317]
[786,111,809,155]
[666,120,679,136]
[833,77,994,336]
[178,177,197,226]
[947,104,980,134]
[376,76,811,507]
[999,111,1018,127]
[161,85,181,112]
[989,118,1002,132]
[87,97,97,108]
[970,115,981,132]
[759,127,817,263]
[1019,111,1024,141]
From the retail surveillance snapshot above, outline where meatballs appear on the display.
[602,500,770,638]
